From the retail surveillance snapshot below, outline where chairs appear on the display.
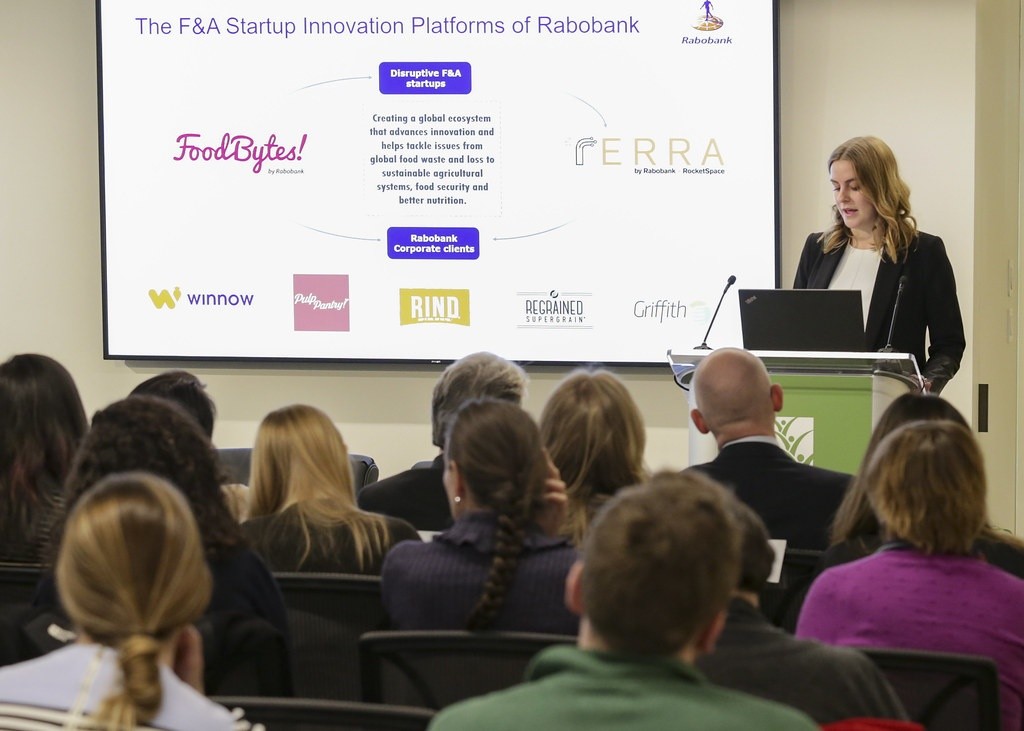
[0,451,1002,728]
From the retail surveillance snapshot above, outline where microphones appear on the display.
[878,275,908,374]
[694,275,737,350]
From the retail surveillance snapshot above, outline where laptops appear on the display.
[738,289,867,351]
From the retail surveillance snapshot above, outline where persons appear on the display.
[791,134,967,397]
[0,348,1024,731]
